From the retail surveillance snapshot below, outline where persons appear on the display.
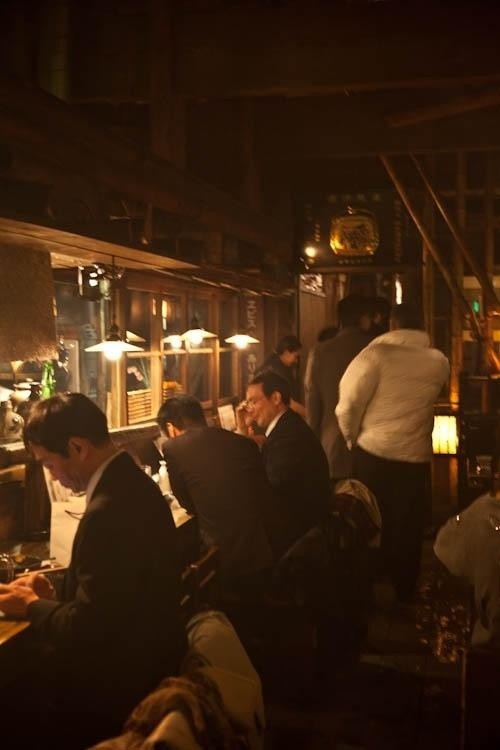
[0,388,189,749]
[303,291,449,600]
[232,370,332,558]
[153,393,276,570]
[253,337,305,414]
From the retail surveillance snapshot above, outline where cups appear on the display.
[140,465,152,477]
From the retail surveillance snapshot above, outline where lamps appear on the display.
[84,251,262,361]
[431,414,461,456]
